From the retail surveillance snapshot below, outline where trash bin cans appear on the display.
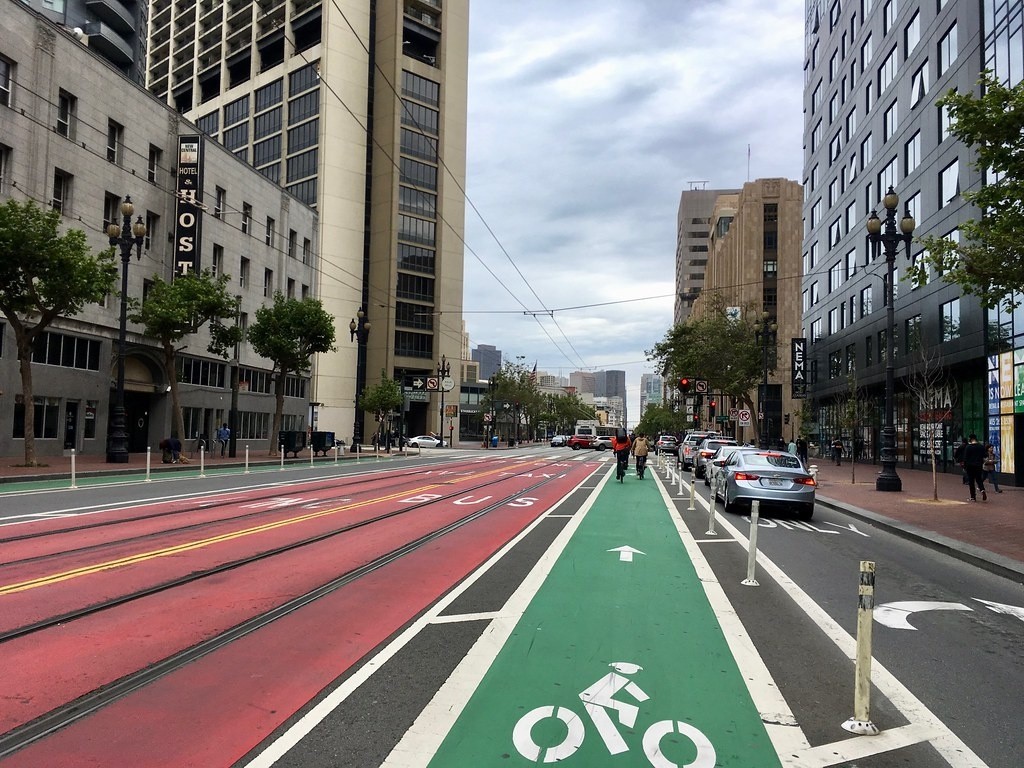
[163,440,178,463]
[311,431,335,452]
[279,431,306,453]
[509,437,514,446]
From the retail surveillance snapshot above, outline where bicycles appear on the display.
[613,448,630,482]
[631,450,650,480]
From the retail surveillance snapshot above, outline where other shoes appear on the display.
[995,490,1002,493]
[616,476,620,479]
[967,498,975,501]
[982,490,987,500]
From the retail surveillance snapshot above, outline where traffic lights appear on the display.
[449,426,453,430]
[784,413,790,425]
[697,394,703,405]
[678,378,691,393]
[709,401,716,418]
[514,400,519,412]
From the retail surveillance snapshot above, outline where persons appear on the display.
[831,437,836,462]
[632,433,650,475]
[788,435,808,464]
[955,438,981,491]
[630,433,637,444]
[962,434,989,502]
[219,423,230,458]
[614,428,631,479]
[834,437,845,466]
[977,444,1002,494]
[777,437,787,451]
[393,427,399,446]
[518,427,523,444]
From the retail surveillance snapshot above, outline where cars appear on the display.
[675,429,760,489]
[713,447,817,522]
[371,431,407,447]
[654,435,679,456]
[406,435,449,448]
[550,408,626,451]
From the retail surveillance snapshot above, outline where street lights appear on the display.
[436,353,451,447]
[488,371,499,445]
[105,195,148,464]
[865,183,917,493]
[753,302,780,450]
[347,306,373,453]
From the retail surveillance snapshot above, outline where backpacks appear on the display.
[616,428,628,444]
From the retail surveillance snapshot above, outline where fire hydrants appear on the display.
[808,463,820,485]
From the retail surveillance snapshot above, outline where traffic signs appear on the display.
[412,377,426,391]
[503,402,511,410]
